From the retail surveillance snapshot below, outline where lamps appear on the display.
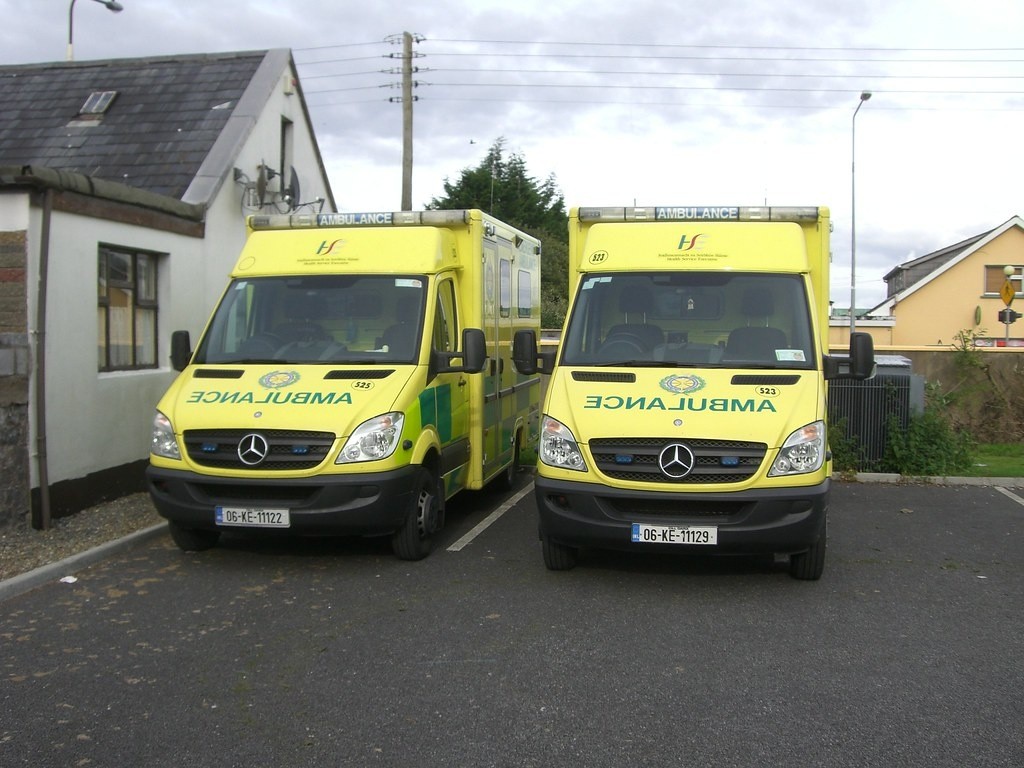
[234,158,327,213]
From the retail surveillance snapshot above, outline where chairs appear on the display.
[375,298,416,355]
[726,292,786,360]
[274,293,321,340]
[600,288,666,365]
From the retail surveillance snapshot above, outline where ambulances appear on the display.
[143,208,544,563]
[510,204,878,581]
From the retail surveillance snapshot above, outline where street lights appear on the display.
[469,139,496,215]
[65,0,125,62]
[1004,266,1014,343]
[850,90,874,333]
